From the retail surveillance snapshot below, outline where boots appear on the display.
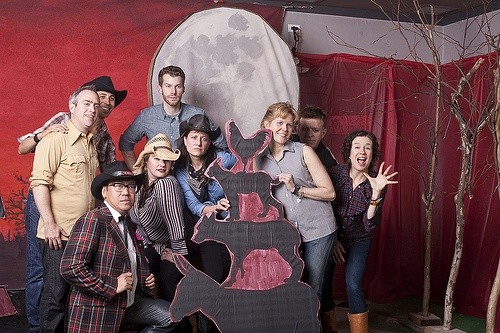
[346,309,370,333]
[320,307,337,333]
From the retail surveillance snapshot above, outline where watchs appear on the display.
[291,184,300,195]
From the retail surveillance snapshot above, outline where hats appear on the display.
[133,133,181,169]
[90,161,146,200]
[79,76,127,108]
[179,114,221,141]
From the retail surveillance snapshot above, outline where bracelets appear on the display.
[370,197,382,207]
[34,133,40,144]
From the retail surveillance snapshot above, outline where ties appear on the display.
[119,215,132,248]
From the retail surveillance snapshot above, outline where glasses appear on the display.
[109,183,138,193]
[302,129,321,133]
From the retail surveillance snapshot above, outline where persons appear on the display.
[17,65,399,333]
